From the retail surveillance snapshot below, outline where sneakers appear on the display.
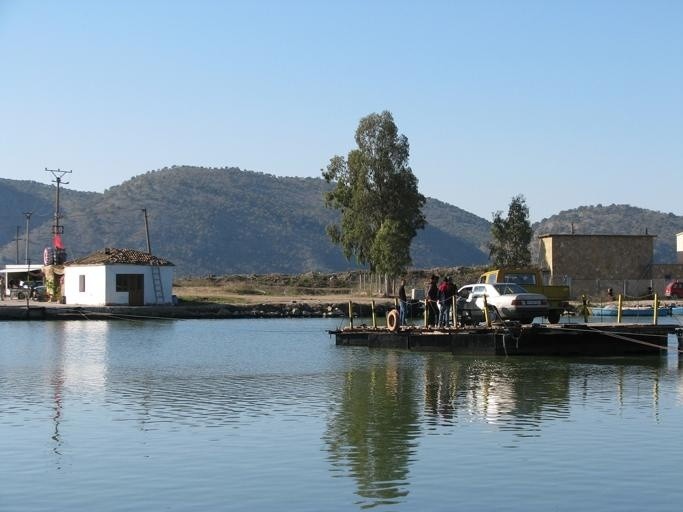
[400,323,408,326]
[427,323,453,330]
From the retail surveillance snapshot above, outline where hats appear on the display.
[444,276,454,282]
[431,274,440,280]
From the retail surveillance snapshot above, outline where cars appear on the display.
[449,283,569,324]
[665,280,683,299]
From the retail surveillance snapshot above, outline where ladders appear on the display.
[152,267,165,305]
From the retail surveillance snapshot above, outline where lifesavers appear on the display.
[387,310,399,332]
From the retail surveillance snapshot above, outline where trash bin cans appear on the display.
[171,295,176,305]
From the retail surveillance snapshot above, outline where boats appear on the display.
[587,305,683,316]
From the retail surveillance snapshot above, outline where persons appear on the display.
[398,279,407,326]
[426,273,439,328]
[435,275,457,327]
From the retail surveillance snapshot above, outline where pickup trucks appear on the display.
[475,267,570,323]
[6,281,43,299]
